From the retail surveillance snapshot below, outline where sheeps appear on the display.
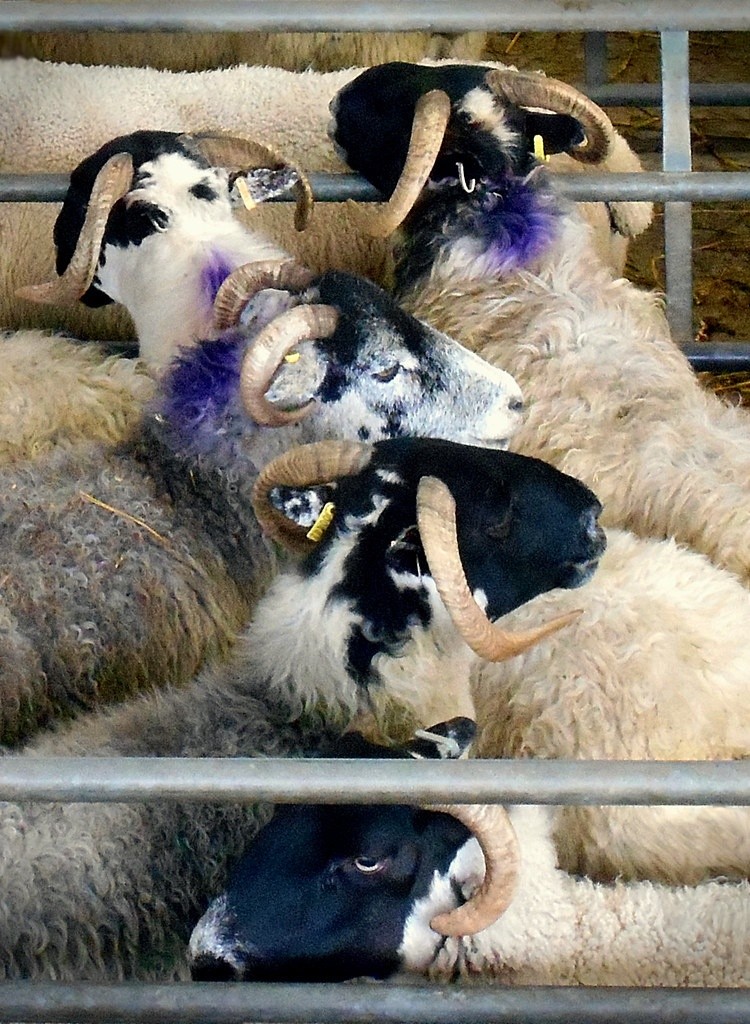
[1,30,515,76]
[0,51,660,359]
[177,713,750,991]
[326,60,750,588]
[0,327,168,467]
[466,522,750,883]
[8,121,316,380]
[2,440,608,986]
[0,256,533,756]
